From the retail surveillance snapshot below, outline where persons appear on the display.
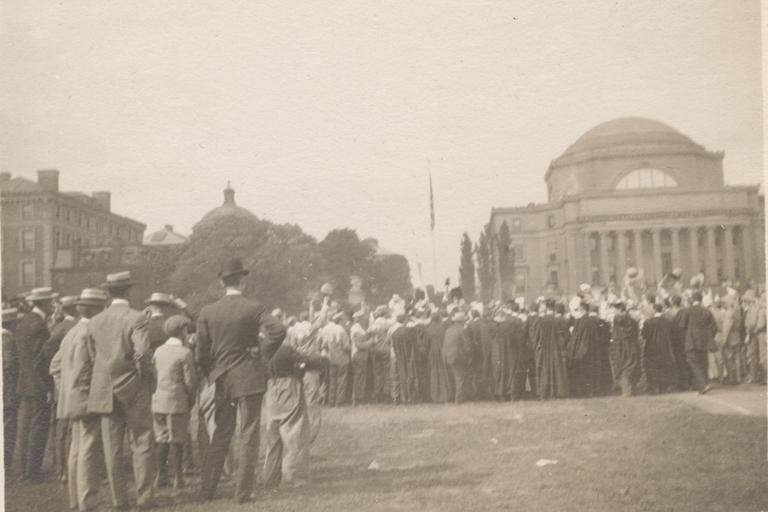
[194,259,287,503]
[299,264,767,406]
[46,271,159,508]
[1,258,327,487]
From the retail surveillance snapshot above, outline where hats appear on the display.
[328,305,389,323]
[613,303,624,310]
[623,266,642,283]
[215,259,248,279]
[24,286,58,301]
[320,284,333,295]
[624,266,706,290]
[451,312,468,322]
[164,315,191,335]
[719,288,766,308]
[60,270,138,308]
[2,308,24,322]
[143,293,171,305]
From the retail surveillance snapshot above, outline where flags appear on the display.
[428,170,436,231]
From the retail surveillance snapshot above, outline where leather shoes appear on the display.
[698,384,711,395]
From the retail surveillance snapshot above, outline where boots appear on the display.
[171,443,185,487]
[155,442,170,487]
